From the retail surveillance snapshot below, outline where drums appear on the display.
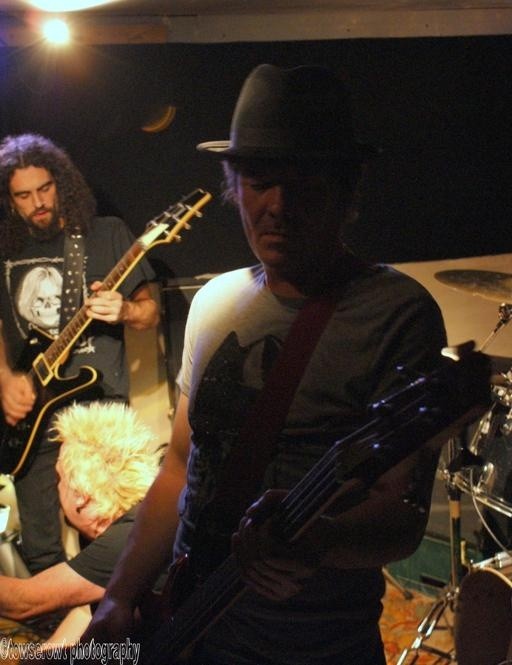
[453,407,512,519]
[467,370,512,462]
[453,548,512,665]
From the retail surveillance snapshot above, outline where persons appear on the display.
[0,64,497,665]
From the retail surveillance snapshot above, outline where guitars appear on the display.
[25,369,459,664]
[0,187,213,480]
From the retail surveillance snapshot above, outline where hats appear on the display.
[196,63,327,159]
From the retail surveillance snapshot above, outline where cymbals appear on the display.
[433,271,512,305]
